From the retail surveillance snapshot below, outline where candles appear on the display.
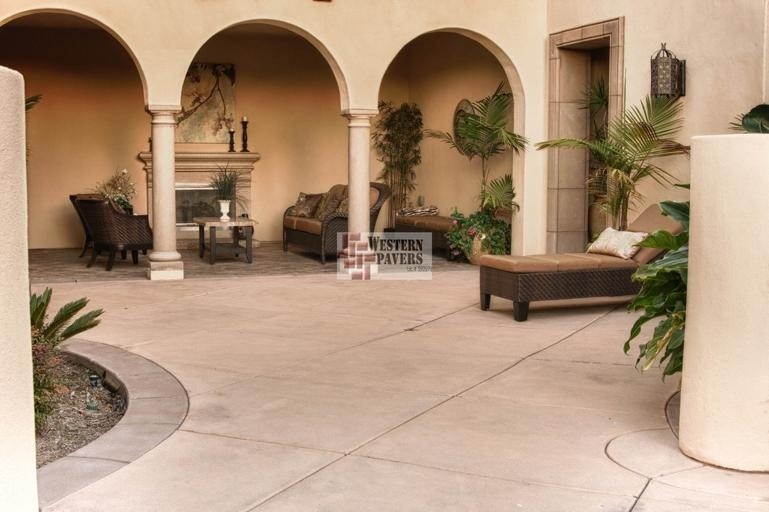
[243,116,248,121]
[230,128,234,131]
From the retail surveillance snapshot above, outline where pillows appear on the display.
[585,225,648,259]
[286,183,347,222]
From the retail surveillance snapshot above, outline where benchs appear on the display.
[396,211,458,251]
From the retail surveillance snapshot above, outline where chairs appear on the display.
[70,192,153,273]
[282,181,390,264]
[480,203,685,321]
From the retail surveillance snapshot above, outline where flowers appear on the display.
[447,208,515,259]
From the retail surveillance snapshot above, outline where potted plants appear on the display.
[207,159,250,220]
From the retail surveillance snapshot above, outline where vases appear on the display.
[462,232,490,264]
